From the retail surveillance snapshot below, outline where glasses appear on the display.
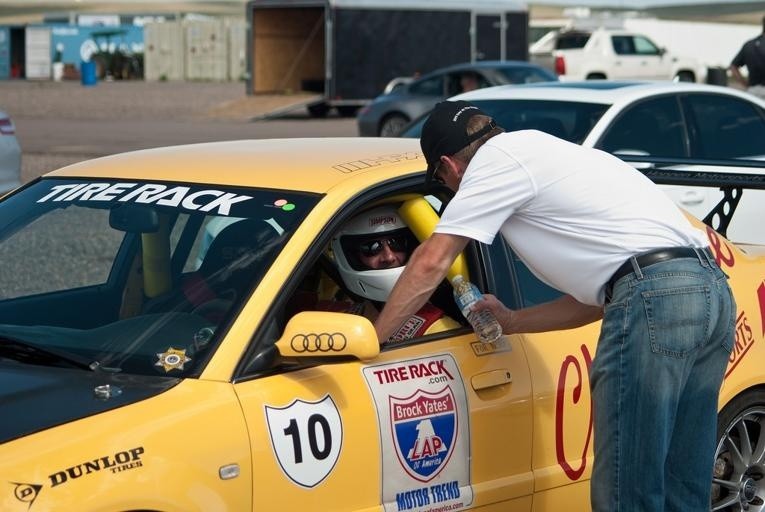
[356,237,410,259]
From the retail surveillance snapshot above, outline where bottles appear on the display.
[451,274,503,344]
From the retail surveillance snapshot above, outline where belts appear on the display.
[608,246,716,293]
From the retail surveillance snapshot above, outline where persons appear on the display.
[731,18,765,100]
[373,100,736,512]
[271,202,461,368]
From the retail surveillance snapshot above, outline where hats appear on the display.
[419,100,498,186]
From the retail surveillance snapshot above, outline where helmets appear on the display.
[327,203,421,303]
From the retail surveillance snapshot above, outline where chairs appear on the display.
[135,218,281,314]
[510,112,765,159]
[461,75,480,93]
